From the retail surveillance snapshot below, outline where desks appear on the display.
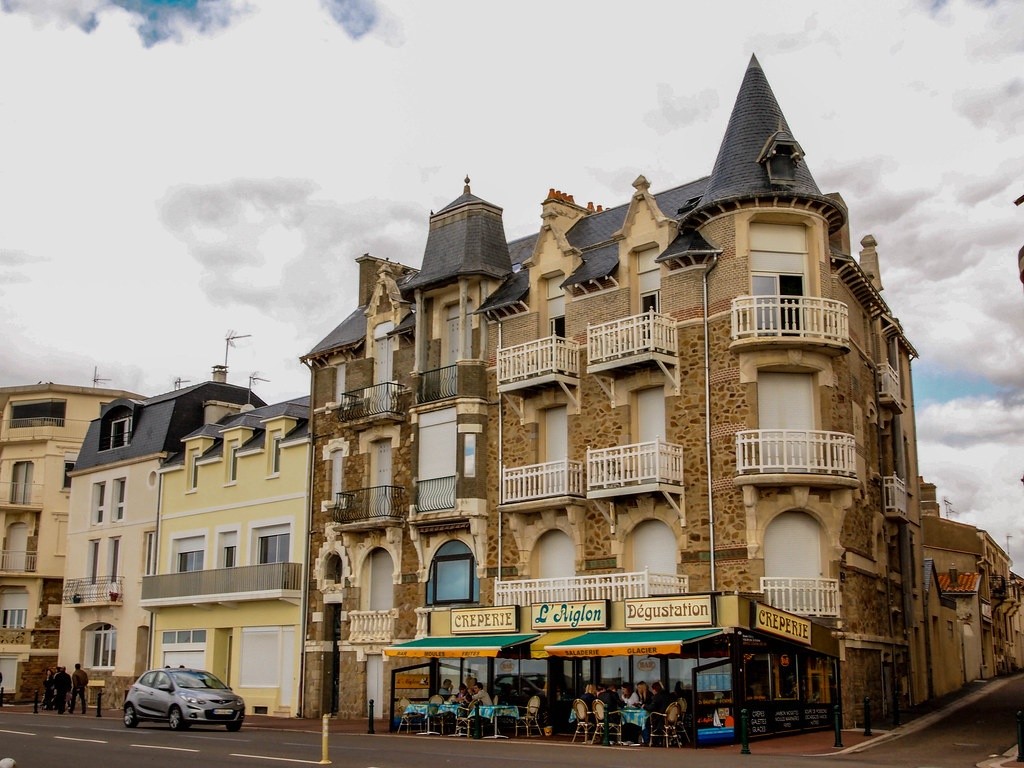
[621,709,649,731]
[467,705,519,739]
[437,704,462,734]
[397,703,444,735]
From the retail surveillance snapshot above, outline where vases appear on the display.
[111,595,117,602]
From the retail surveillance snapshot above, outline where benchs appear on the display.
[591,699,623,746]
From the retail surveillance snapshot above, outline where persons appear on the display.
[39,663,88,715]
[159,665,185,685]
[580,679,672,747]
[438,676,492,706]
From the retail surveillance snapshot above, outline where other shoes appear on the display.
[68,709,73,714]
[623,741,634,746]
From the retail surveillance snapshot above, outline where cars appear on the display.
[124,668,245,731]
[493,673,572,703]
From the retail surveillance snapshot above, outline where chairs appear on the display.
[649,702,682,749]
[514,696,542,737]
[428,694,444,704]
[571,698,594,745]
[396,696,423,734]
[676,698,690,743]
[455,698,484,738]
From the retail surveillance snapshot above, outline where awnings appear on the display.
[530,631,588,659]
[383,632,547,658]
[543,628,723,657]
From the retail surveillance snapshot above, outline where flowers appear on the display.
[109,591,119,596]
